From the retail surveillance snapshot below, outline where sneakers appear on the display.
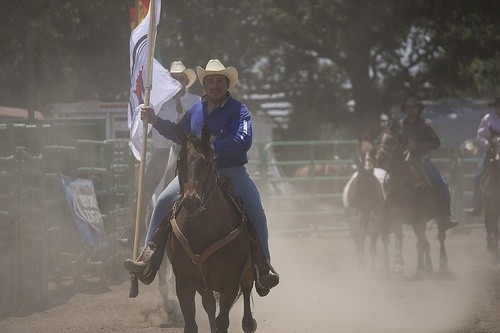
[255,274,279,297]
[124,259,156,285]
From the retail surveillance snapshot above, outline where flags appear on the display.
[126,0,181,160]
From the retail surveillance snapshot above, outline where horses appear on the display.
[163,120,259,333]
[477,122,500,268]
[370,117,452,282]
[340,136,386,270]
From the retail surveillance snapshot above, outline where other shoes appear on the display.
[464,207,482,216]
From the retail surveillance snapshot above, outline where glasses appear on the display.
[173,77,186,81]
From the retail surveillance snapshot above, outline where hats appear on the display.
[196,59,238,91]
[488,98,500,108]
[170,61,197,89]
[400,97,424,115]
[460,139,479,157]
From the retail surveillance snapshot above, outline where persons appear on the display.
[123,58,279,297]
[394,96,458,229]
[463,95,500,215]
[353,111,399,176]
